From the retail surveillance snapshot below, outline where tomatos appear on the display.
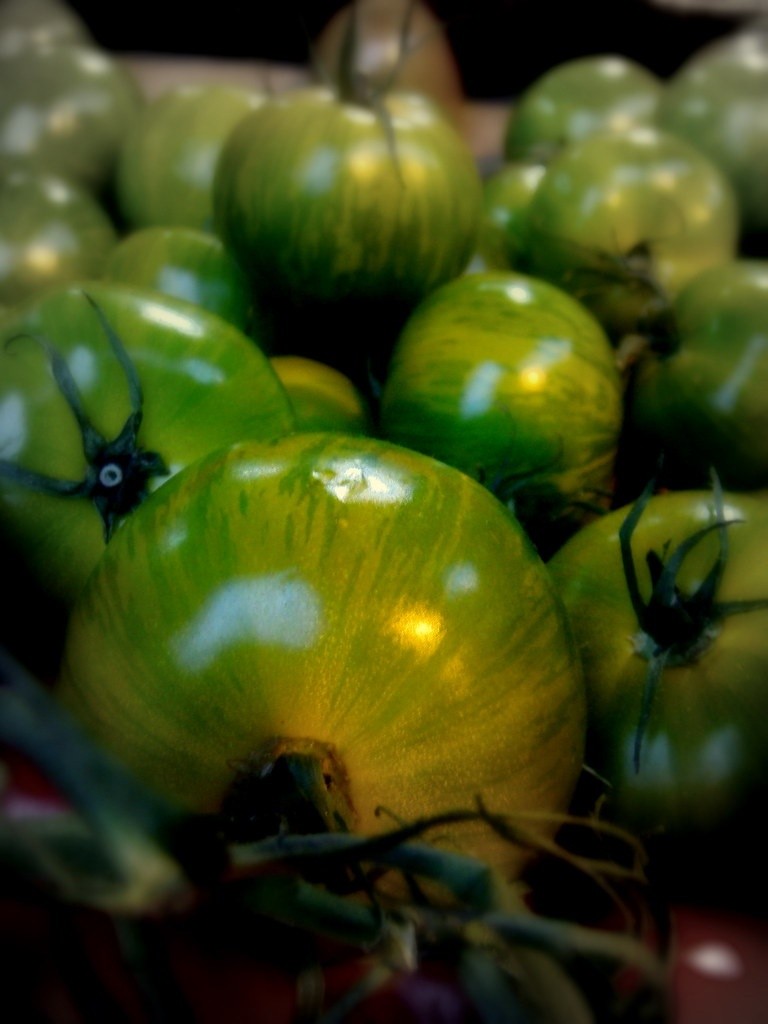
[0,0,768,1024]
